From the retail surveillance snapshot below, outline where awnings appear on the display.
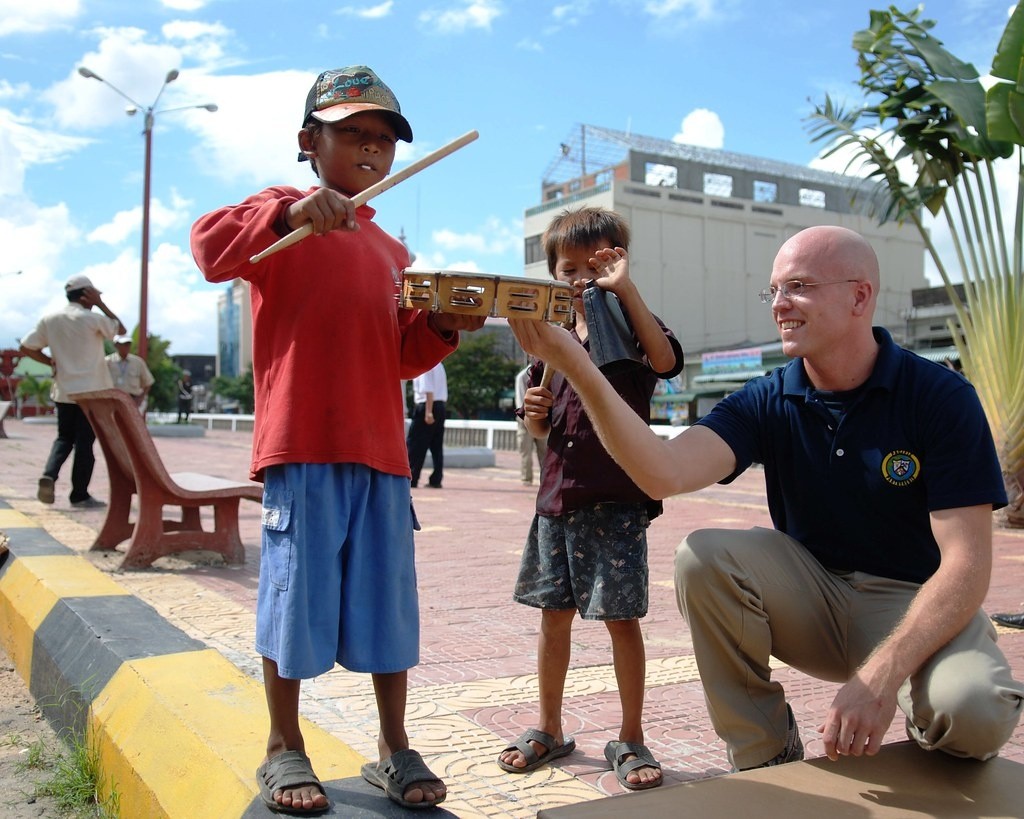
[915,352,960,362]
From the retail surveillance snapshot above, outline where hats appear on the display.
[64,279,102,296]
[112,332,132,344]
[301,66,413,144]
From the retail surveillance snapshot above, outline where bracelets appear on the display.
[425,412,433,417]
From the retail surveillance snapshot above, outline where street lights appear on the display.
[397,226,417,419]
[75,64,219,420]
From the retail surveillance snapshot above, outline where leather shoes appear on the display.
[990,610,1024,629]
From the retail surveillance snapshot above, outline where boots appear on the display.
[257,751,331,814]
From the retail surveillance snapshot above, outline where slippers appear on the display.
[498,727,575,774]
[604,738,662,790]
[360,749,446,807]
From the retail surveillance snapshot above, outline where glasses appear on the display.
[758,279,864,303]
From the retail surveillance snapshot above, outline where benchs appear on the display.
[66,387,267,574]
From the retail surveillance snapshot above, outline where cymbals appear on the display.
[392,270,576,324]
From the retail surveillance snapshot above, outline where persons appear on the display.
[499,205,683,790]
[190,66,488,816]
[173,369,194,424]
[507,225,1024,774]
[19,274,128,508]
[92,334,156,423]
[514,353,551,486]
[406,362,448,488]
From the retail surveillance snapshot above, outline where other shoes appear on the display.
[70,496,108,511]
[424,480,443,489]
[728,703,805,776]
[39,476,54,503]
